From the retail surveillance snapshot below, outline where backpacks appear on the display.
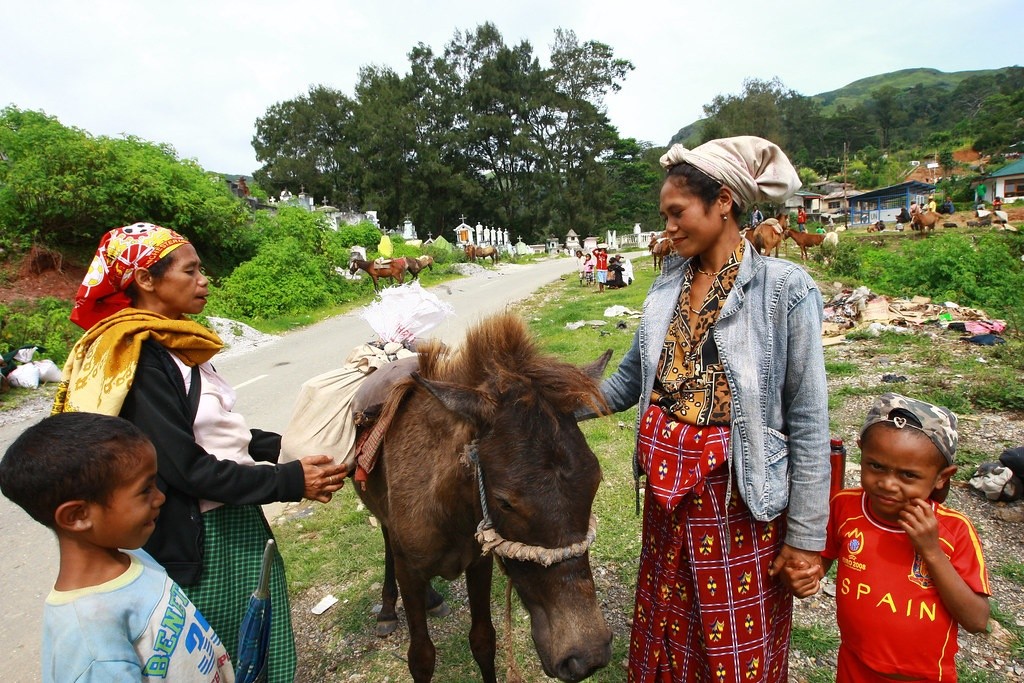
[606,265,619,281]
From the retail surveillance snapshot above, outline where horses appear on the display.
[463,244,498,265]
[910,207,938,231]
[745,211,790,258]
[403,255,434,281]
[349,313,615,683]
[649,233,676,271]
[350,255,406,290]
[784,228,825,260]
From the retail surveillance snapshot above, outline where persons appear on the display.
[48,220,347,683]
[750,205,833,234]
[992,197,1003,210]
[942,196,955,214]
[976,180,987,204]
[0,412,236,683]
[575,248,635,293]
[976,201,985,215]
[779,391,991,683]
[570,134,830,682]
[875,194,936,233]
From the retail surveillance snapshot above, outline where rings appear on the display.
[329,476,332,485]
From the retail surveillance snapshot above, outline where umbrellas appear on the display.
[233,537,274,683]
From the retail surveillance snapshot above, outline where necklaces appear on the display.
[690,307,700,315]
[697,265,718,276]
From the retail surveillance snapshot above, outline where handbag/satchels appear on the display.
[968,463,1013,500]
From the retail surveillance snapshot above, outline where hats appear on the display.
[859,392,958,504]
